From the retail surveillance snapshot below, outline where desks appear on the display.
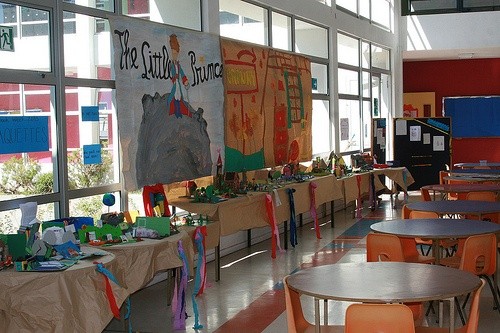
[168,173,344,283]
[334,166,415,220]
[0,219,221,333]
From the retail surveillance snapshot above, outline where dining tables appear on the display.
[284,162,500,333]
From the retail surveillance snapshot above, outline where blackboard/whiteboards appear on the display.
[443,97,500,137]
[394,118,451,189]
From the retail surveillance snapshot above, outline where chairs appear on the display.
[283,168,500,333]
